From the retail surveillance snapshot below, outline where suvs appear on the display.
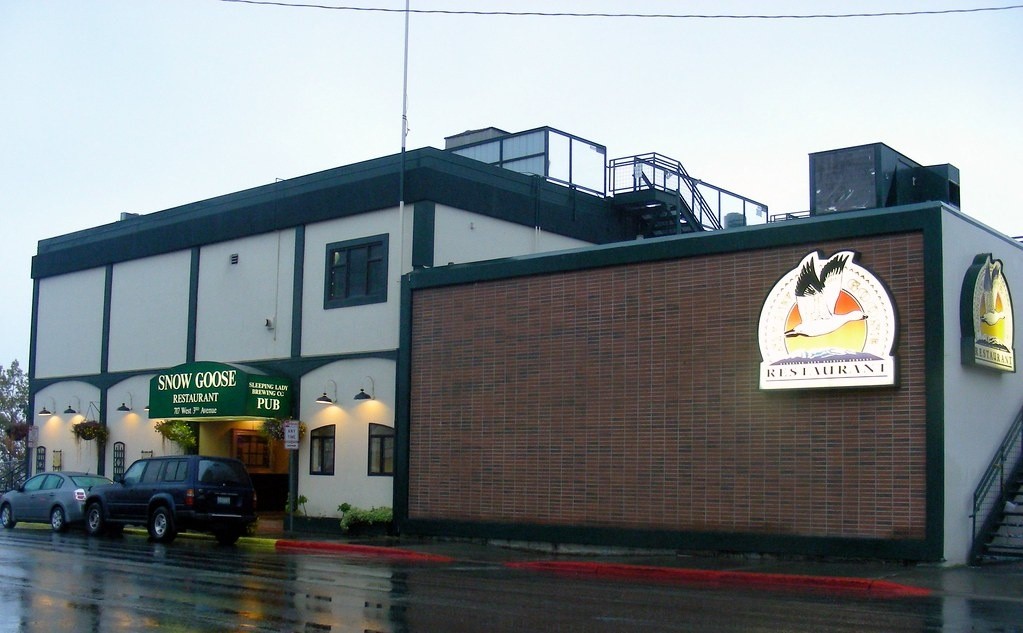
[80,453,261,546]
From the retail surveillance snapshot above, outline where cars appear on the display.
[0,471,116,533]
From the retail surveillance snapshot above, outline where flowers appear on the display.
[261,419,308,440]
[73,421,110,444]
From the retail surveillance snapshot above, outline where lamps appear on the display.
[64,395,80,413]
[353,376,376,401]
[117,392,132,412]
[315,380,339,406]
[144,406,149,410]
[37,396,56,415]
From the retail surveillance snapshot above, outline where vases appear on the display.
[273,433,284,441]
[81,431,96,440]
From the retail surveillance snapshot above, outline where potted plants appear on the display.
[154,420,196,448]
[338,502,396,536]
[6,422,32,450]
